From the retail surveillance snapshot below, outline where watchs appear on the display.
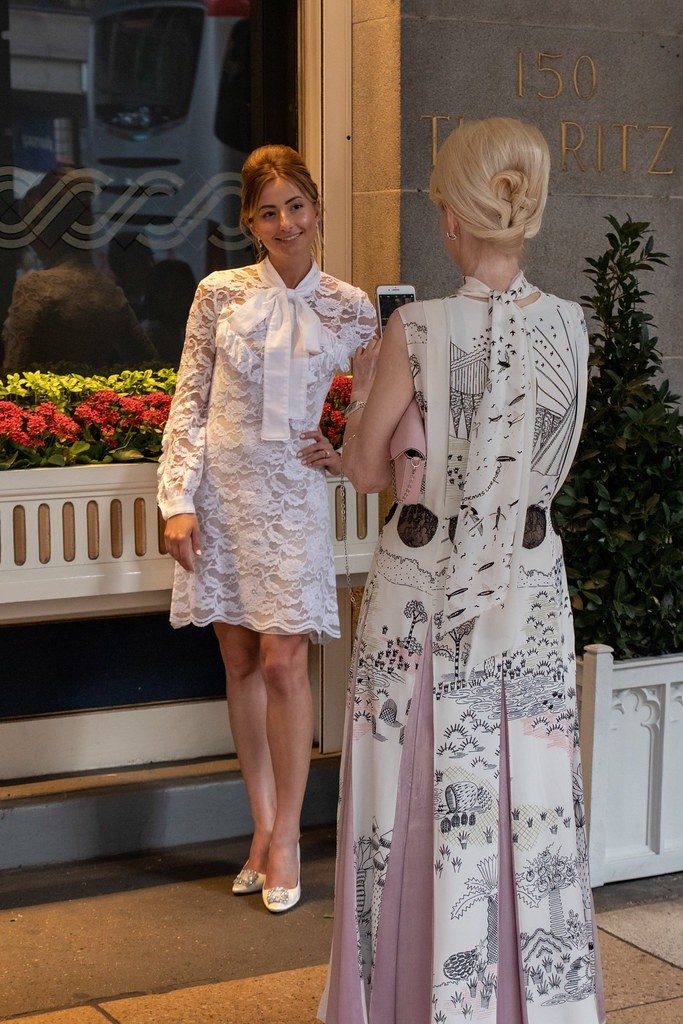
[344,400,366,418]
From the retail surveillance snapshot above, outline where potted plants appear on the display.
[541,225,683,891]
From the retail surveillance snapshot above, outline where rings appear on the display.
[324,450,330,457]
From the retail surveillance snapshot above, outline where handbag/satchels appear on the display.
[391,400,426,505]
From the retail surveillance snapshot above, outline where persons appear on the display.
[3,166,158,368]
[318,116,607,1024]
[155,143,378,912]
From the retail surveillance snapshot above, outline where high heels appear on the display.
[232,859,265,894]
[263,841,300,912]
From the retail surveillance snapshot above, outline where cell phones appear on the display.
[374,284,415,339]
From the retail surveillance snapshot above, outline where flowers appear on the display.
[0,368,354,471]
